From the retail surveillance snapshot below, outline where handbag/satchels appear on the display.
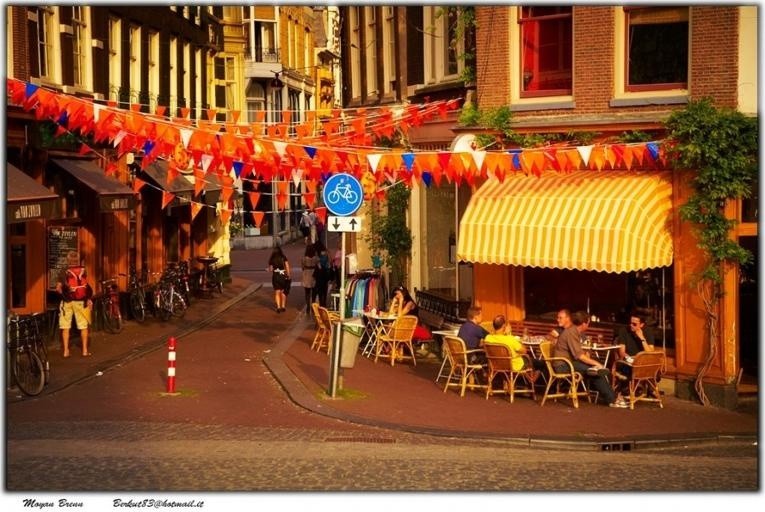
[283,279,291,296]
[312,264,320,281]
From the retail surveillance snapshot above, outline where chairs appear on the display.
[443,336,665,410]
[310,302,419,368]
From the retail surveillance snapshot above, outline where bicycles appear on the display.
[9,306,52,398]
[98,254,226,335]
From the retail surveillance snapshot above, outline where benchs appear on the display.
[414,309,444,348]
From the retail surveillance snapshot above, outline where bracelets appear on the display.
[641,339,646,341]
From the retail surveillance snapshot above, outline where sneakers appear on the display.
[609,398,631,409]
[277,308,286,313]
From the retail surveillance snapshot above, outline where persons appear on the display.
[299,202,343,314]
[484,314,550,383]
[545,307,572,345]
[550,310,630,409]
[55,250,94,359]
[264,245,291,314]
[457,305,491,379]
[384,285,423,355]
[614,311,659,400]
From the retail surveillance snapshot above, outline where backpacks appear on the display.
[62,265,88,299]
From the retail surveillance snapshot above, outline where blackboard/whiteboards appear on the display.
[46,225,80,291]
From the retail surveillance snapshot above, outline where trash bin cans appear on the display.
[340,319,364,367]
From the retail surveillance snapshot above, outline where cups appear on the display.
[517,328,605,349]
[362,302,386,317]
